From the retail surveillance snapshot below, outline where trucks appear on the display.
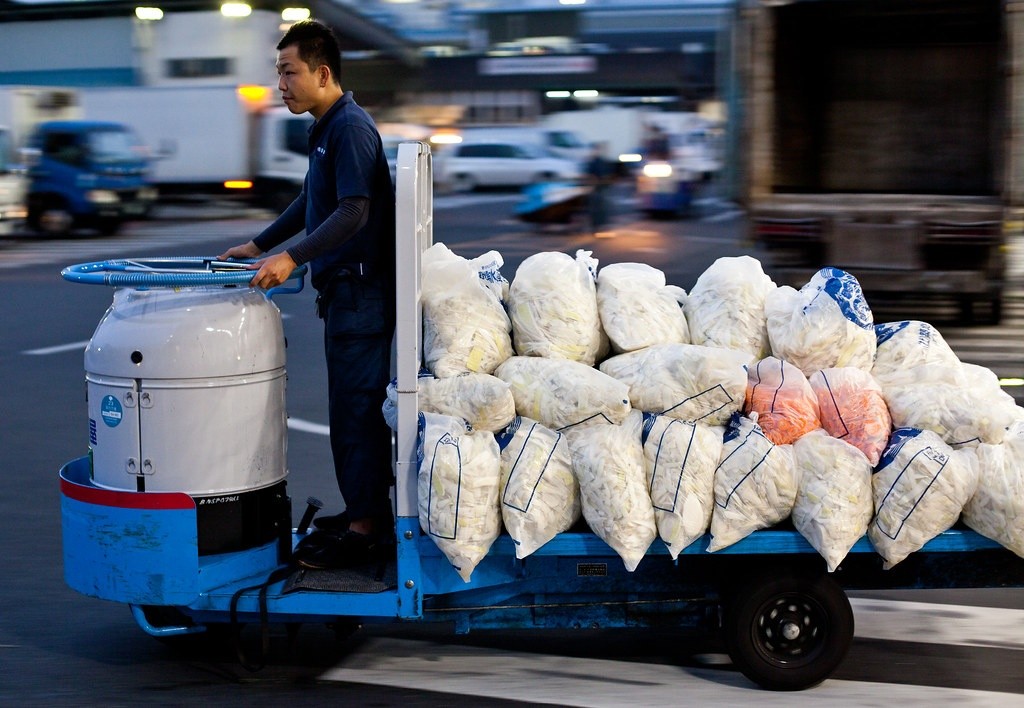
[0,85,161,239]
[0,78,430,219]
[728,1,1023,331]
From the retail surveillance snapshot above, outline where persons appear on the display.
[578,142,613,228]
[14,90,133,237]
[647,124,670,159]
[215,20,395,569]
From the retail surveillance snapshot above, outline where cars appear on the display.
[430,107,723,196]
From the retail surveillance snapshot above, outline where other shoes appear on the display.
[295,499,397,570]
